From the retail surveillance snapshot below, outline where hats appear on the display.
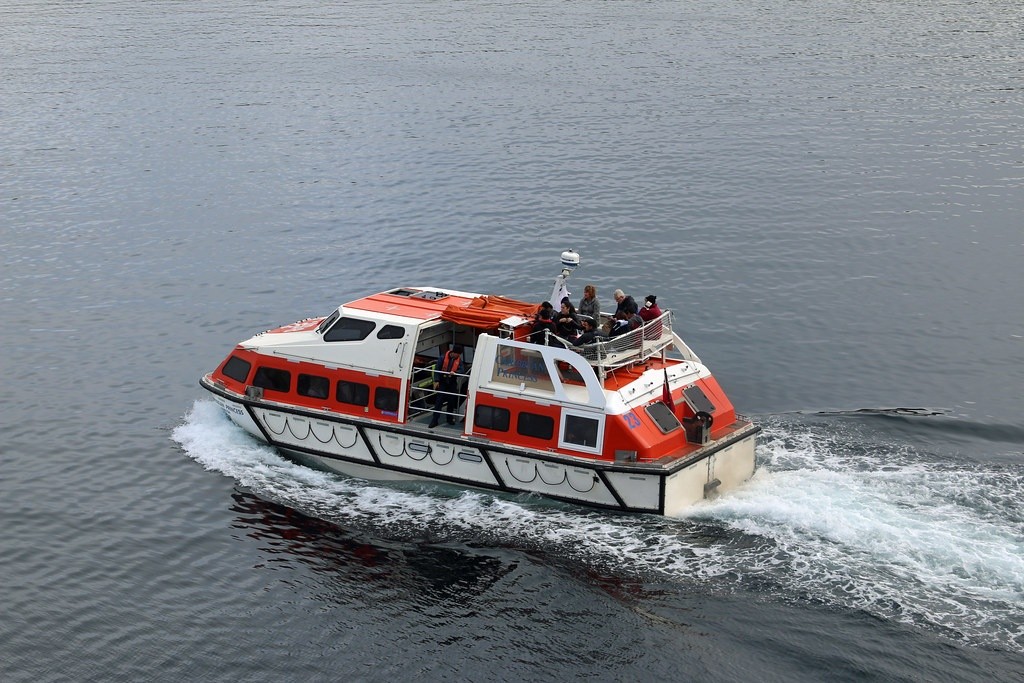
[646,295,656,305]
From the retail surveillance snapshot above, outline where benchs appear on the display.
[520,323,673,389]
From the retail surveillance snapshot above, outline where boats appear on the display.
[198,247,765,517]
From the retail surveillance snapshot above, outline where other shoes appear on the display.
[446,419,456,426]
[429,419,439,428]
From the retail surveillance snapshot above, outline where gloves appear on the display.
[646,301,652,308]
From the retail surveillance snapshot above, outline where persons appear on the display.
[614,289,638,315]
[604,306,642,351]
[579,285,600,327]
[640,295,662,339]
[531,297,585,348]
[573,319,606,359]
[429,343,464,428]
